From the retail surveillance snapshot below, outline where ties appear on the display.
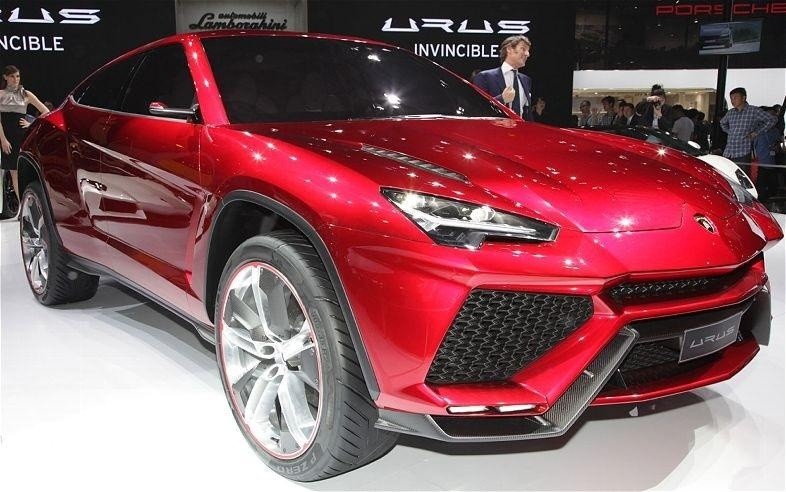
[510,69,521,118]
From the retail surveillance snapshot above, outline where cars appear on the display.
[14,34,785,484]
[698,23,732,50]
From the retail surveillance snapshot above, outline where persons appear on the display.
[34,100,55,116]
[472,36,545,122]
[0,65,50,204]
[577,84,786,215]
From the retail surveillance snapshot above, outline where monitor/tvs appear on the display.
[697,16,767,58]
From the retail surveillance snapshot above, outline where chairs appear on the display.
[229,70,345,122]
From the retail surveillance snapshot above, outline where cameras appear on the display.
[653,100,663,110]
